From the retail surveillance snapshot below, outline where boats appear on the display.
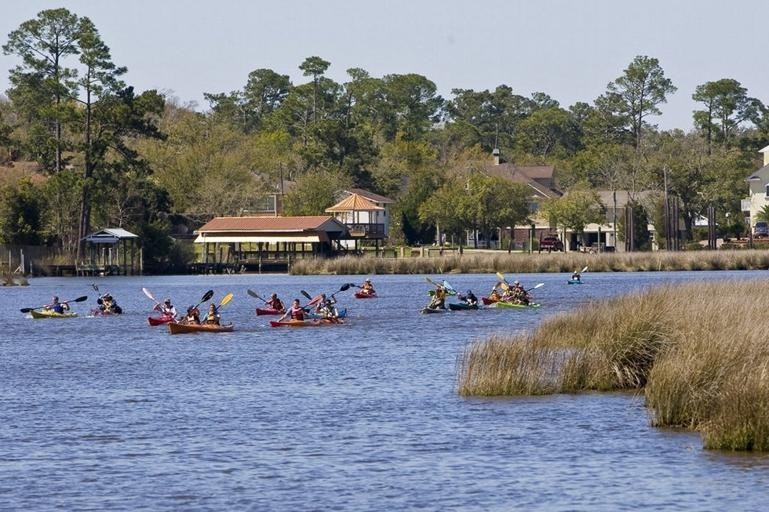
[147,314,176,327]
[89,308,118,316]
[29,308,77,319]
[168,321,235,334]
[567,279,585,285]
[421,289,540,313]
[255,306,348,328]
[354,291,376,298]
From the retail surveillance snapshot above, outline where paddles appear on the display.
[298,295,323,311]
[496,272,510,286]
[326,283,349,300]
[20,296,87,313]
[579,266,588,276]
[181,290,213,322]
[351,283,375,293]
[489,282,500,298]
[525,283,544,292]
[301,290,334,319]
[425,277,441,289]
[201,294,233,323]
[444,281,459,294]
[247,289,271,306]
[143,287,180,323]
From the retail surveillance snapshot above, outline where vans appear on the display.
[752,221,769,239]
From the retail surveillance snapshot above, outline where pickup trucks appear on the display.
[540,235,564,252]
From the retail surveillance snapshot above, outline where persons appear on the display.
[43,295,70,315]
[359,278,374,295]
[200,303,221,325]
[318,293,337,311]
[153,298,177,320]
[431,279,532,311]
[571,269,582,281]
[96,291,123,315]
[281,298,308,321]
[264,292,286,314]
[181,305,202,324]
[314,298,335,317]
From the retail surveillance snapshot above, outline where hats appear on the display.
[164,298,170,302]
